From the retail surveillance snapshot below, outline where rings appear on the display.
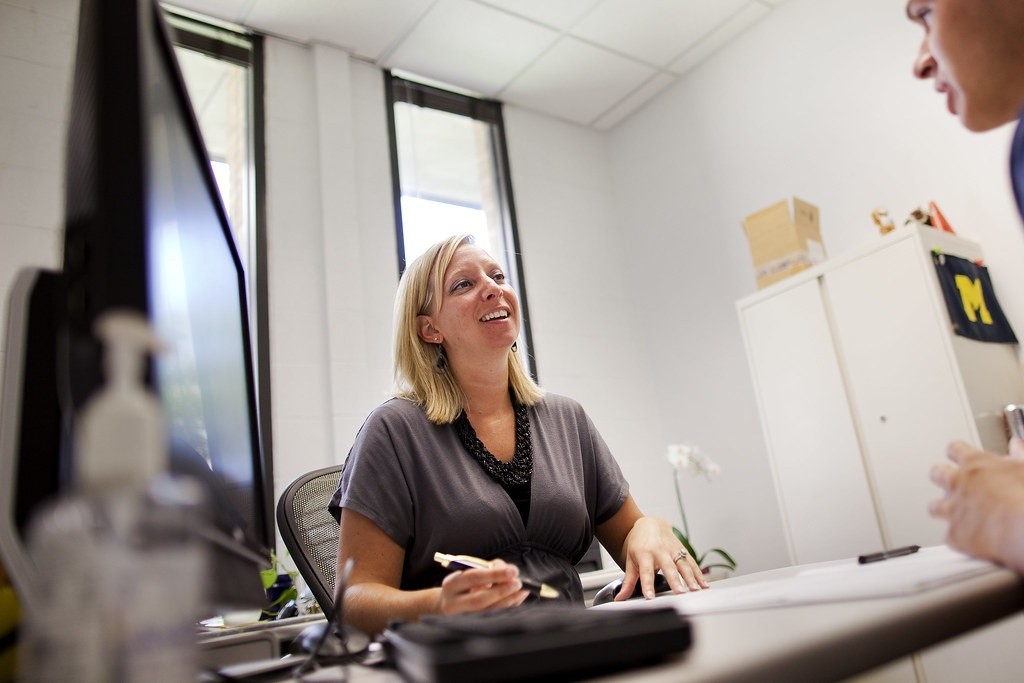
[673,549,689,564]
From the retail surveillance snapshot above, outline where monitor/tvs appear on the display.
[0,0,276,683]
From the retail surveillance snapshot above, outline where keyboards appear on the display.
[379,601,693,683]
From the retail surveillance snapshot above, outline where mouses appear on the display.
[290,623,372,668]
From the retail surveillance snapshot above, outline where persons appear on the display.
[908,0,1024,580]
[326,234,712,643]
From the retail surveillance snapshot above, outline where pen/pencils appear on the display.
[433,552,559,599]
[857,544,921,563]
